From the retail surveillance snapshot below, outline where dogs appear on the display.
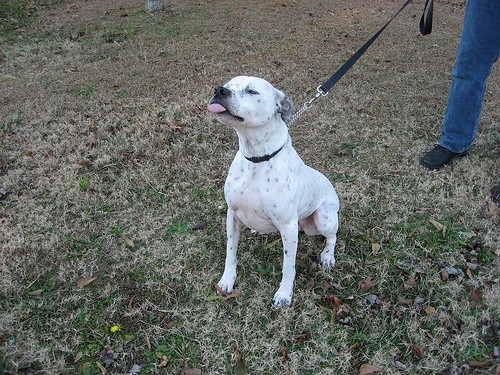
[207,75,343,309]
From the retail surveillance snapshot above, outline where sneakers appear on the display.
[419,143,469,169]
[490,184,500,206]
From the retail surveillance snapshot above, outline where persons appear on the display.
[419,0,500,170]
